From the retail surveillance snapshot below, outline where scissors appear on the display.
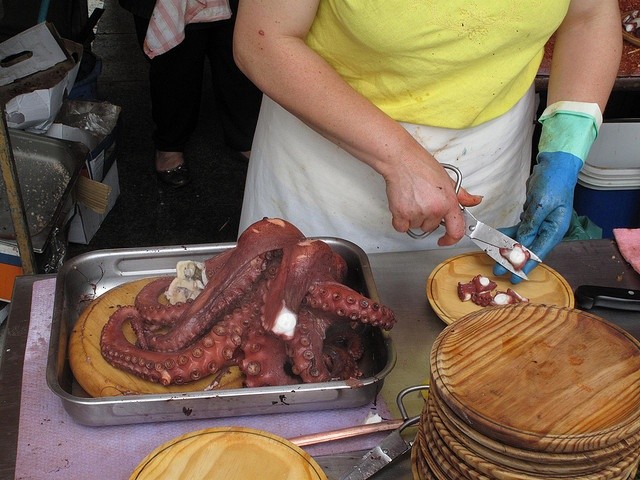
[336,384,431,480]
[405,163,542,281]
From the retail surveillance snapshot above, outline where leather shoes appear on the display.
[153,144,192,188]
[231,151,250,163]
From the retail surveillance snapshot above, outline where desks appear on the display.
[0,238,636,479]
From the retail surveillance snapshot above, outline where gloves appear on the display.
[490,101,605,285]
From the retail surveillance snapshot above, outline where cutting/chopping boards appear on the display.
[410,302,640,480]
[66,275,243,398]
[128,426,328,480]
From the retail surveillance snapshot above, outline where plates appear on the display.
[426,250,576,326]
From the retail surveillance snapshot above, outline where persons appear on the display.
[233,1,622,285]
[126,1,260,206]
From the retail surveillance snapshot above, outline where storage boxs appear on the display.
[574,123,640,237]
